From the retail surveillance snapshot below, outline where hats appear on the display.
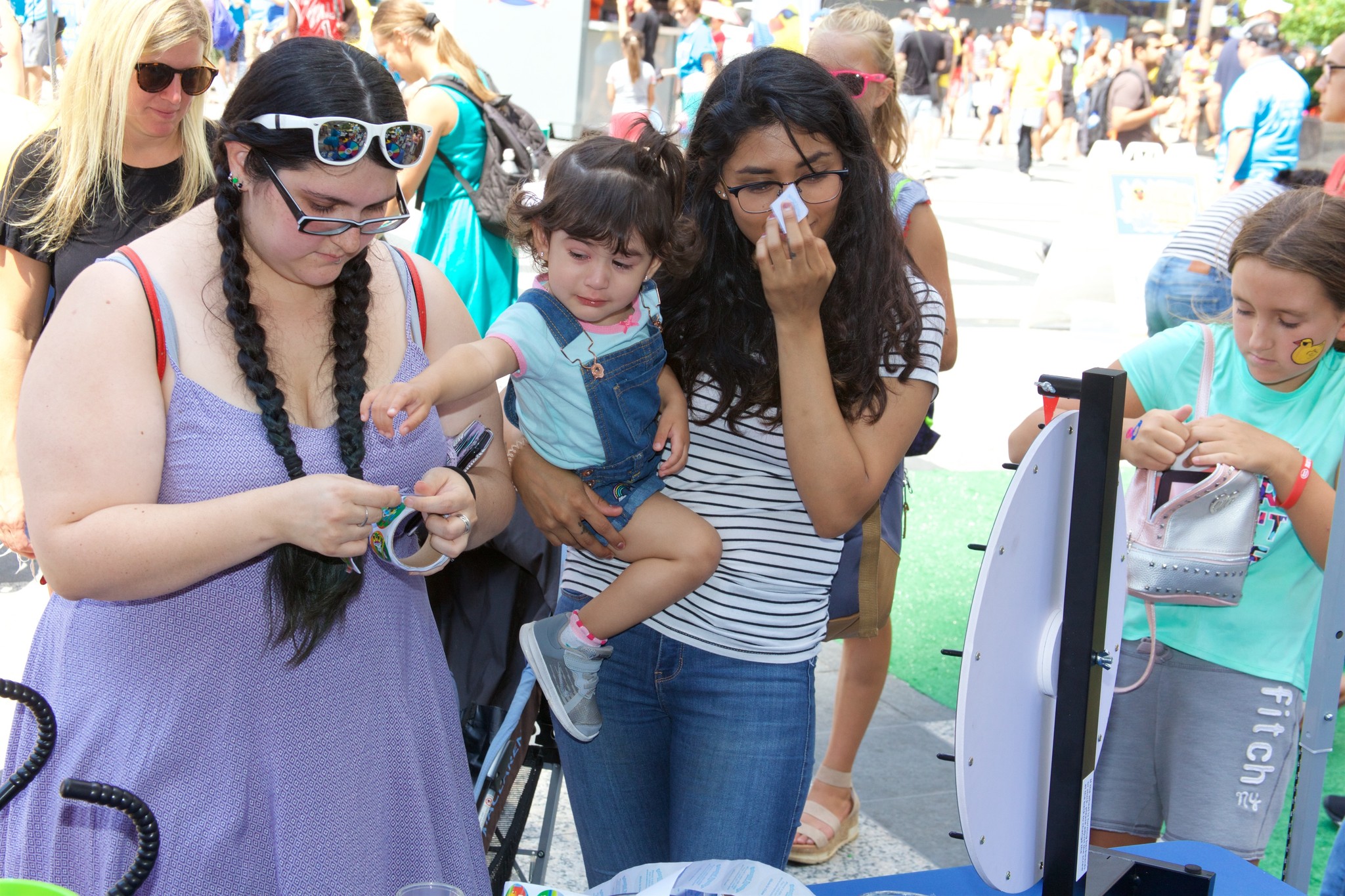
[917,6,932,19]
[1028,11,1046,32]
[1230,18,1280,49]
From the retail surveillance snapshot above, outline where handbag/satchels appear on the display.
[927,71,942,103]
[1125,440,1259,606]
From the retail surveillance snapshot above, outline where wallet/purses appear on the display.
[405,420,494,536]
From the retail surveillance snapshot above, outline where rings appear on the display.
[358,506,368,527]
[454,513,472,535]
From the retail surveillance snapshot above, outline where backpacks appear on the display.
[475,93,552,229]
[1076,75,1113,157]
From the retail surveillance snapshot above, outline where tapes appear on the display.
[369,497,449,572]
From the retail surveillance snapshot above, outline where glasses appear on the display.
[718,167,849,214]
[669,7,687,17]
[257,155,410,236]
[250,114,433,169]
[133,56,219,95]
[827,70,887,98]
[1322,63,1345,84]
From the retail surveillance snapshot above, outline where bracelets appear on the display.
[1273,455,1313,510]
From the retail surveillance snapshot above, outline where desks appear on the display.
[807,840,1304,896]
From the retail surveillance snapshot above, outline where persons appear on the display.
[892,3,1315,193]
[203,0,362,105]
[360,127,722,746]
[372,2,521,341]
[0,0,229,598]
[5,39,518,896]
[506,50,946,884]
[1006,190,1345,863]
[3,1,68,101]
[1143,169,1327,336]
[1314,32,1344,355]
[602,1,805,143]
[787,9,958,863]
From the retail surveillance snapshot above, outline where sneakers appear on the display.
[519,611,614,742]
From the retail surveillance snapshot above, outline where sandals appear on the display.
[788,764,860,865]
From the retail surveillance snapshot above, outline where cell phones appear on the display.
[404,429,493,535]
